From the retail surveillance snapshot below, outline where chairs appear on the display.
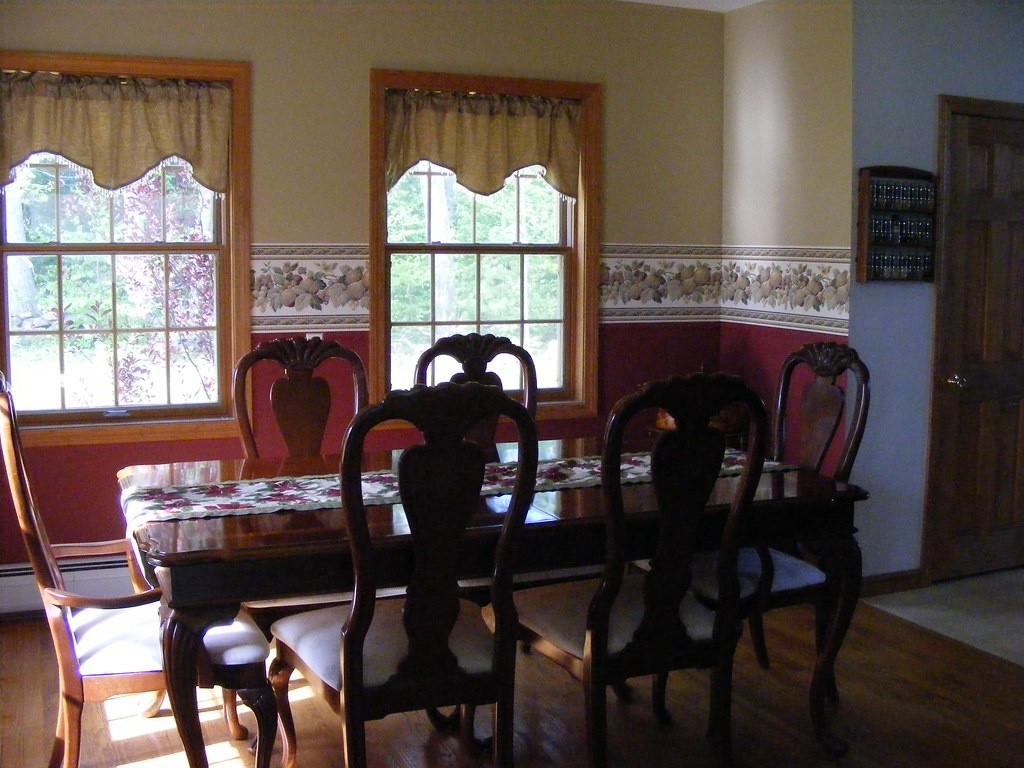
[648,340,872,705]
[264,382,539,768]
[415,331,537,423]
[0,370,279,768]
[234,339,368,640]
[479,372,773,767]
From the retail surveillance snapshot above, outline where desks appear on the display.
[117,449,870,768]
